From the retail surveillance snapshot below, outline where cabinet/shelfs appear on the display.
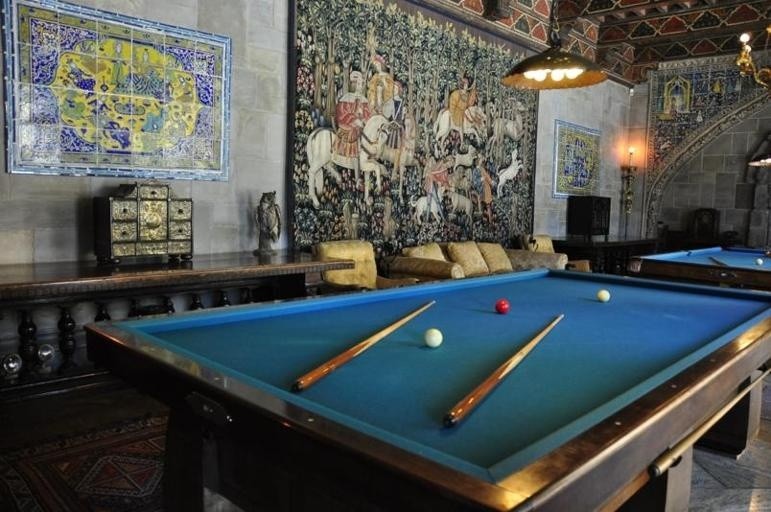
[89,178,193,261]
[565,196,612,244]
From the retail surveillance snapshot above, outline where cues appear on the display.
[294,301,438,391]
[709,256,727,265]
[443,314,565,432]
[648,366,771,481]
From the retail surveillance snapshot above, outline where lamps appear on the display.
[736,24,770,91]
[499,0,607,98]
[747,127,771,169]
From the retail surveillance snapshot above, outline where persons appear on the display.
[331,55,499,225]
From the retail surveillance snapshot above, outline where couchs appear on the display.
[379,241,570,283]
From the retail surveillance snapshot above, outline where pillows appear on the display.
[477,241,515,272]
[400,243,448,264]
[446,239,489,278]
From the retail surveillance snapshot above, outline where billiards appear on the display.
[756,259,764,266]
[496,298,510,314]
[425,329,443,347]
[598,291,611,304]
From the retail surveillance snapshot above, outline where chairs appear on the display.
[687,208,721,244]
[516,234,593,274]
[313,238,417,292]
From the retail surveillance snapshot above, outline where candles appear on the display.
[628,148,633,166]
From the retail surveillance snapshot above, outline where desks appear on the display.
[549,234,656,277]
[628,244,771,292]
[82,266,771,511]
[0,247,353,409]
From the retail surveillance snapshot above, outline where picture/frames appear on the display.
[2,1,233,180]
[286,0,541,254]
[550,118,602,204]
